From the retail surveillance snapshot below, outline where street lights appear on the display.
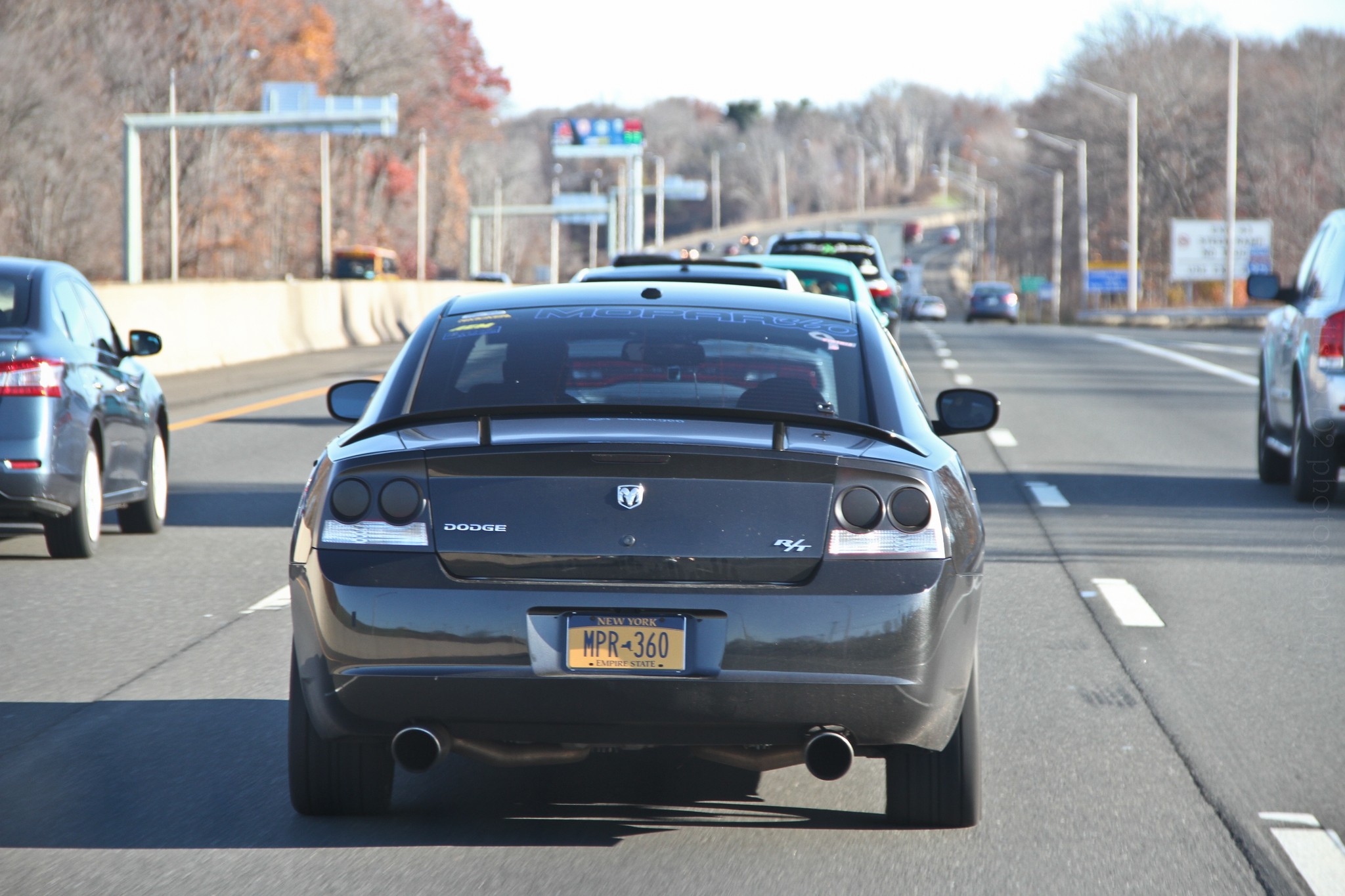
[1011,4,1238,312]
[931,152,998,262]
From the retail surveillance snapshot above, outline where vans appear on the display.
[331,247,408,282]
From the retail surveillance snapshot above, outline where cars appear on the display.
[475,272,513,285]
[573,213,963,322]
[1,256,169,561]
[970,281,1018,321]
[290,276,1002,828]
[1245,205,1345,499]
